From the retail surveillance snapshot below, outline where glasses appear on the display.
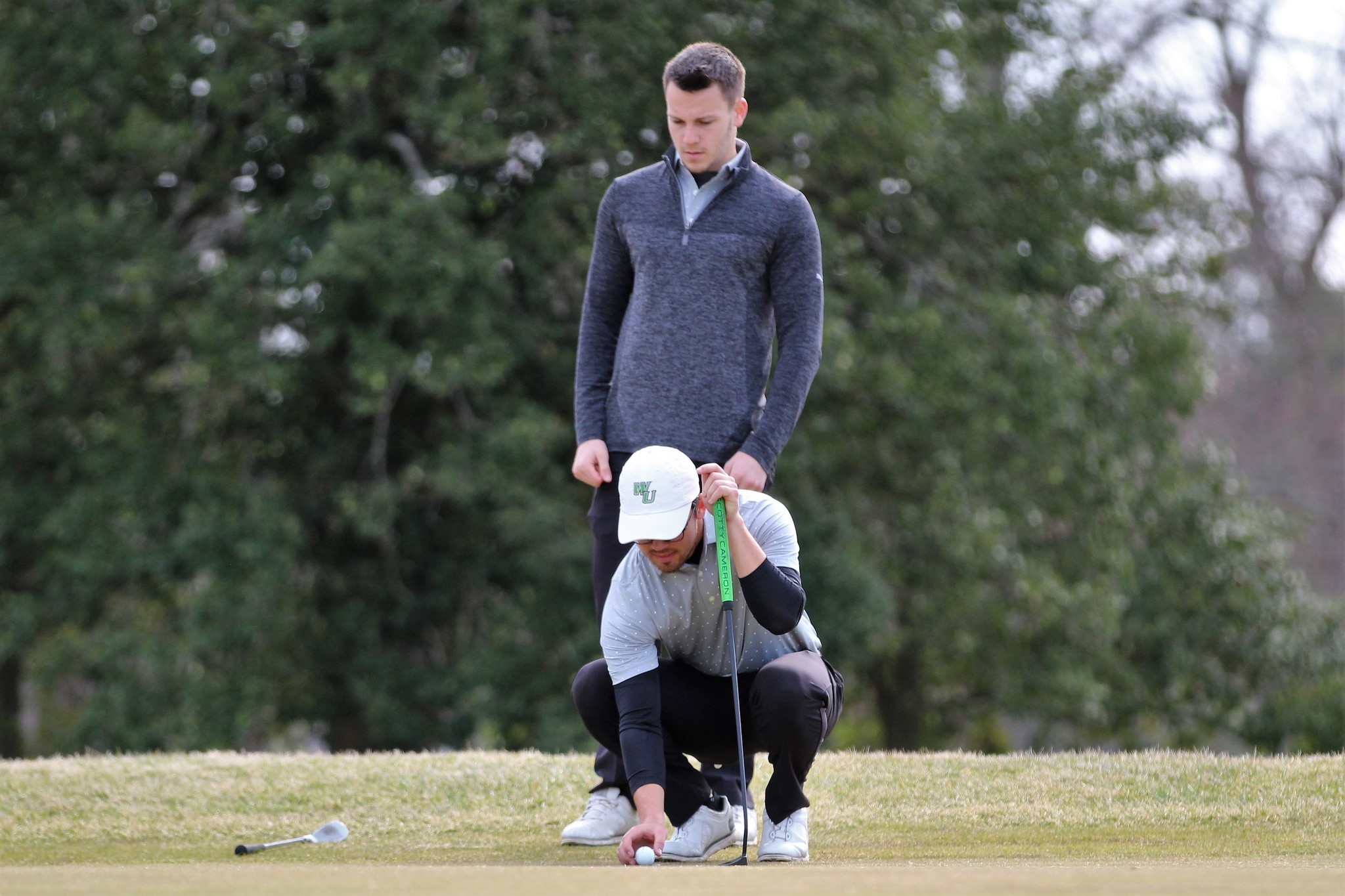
[633,507,695,544]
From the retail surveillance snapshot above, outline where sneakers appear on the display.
[560,787,637,846]
[653,796,737,861]
[731,804,758,846]
[757,807,809,863]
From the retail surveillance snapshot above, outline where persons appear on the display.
[560,40,820,843]
[572,446,844,867]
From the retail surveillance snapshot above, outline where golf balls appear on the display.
[635,845,656,866]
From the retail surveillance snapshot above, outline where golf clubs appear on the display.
[711,496,750,867]
[234,819,350,857]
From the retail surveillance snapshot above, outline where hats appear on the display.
[617,445,700,544]
